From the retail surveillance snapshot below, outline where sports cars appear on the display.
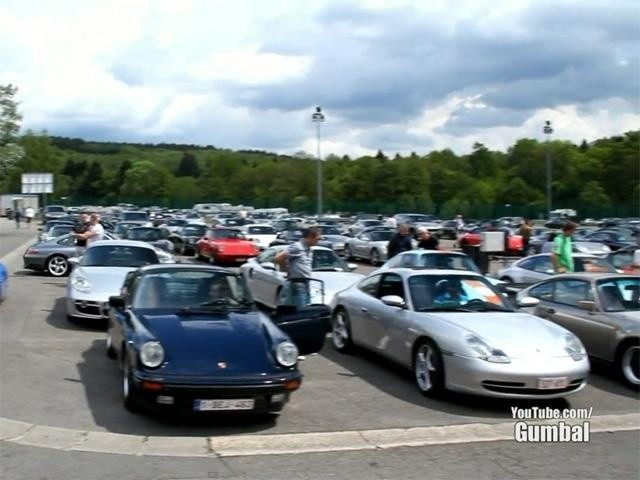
[496,272,640,393]
[326,267,590,406]
[104,261,333,429]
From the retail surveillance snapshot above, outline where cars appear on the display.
[21,202,640,325]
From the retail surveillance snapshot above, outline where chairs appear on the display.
[143,277,168,304]
[196,277,220,304]
[409,285,432,307]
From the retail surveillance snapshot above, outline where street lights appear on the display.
[311,107,326,213]
[544,119,554,213]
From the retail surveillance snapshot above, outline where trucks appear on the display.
[0,193,40,222]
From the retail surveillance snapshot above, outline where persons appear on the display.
[384,215,397,226]
[275,228,320,305]
[387,222,442,259]
[24,206,35,226]
[551,222,579,273]
[519,218,534,251]
[69,212,104,253]
